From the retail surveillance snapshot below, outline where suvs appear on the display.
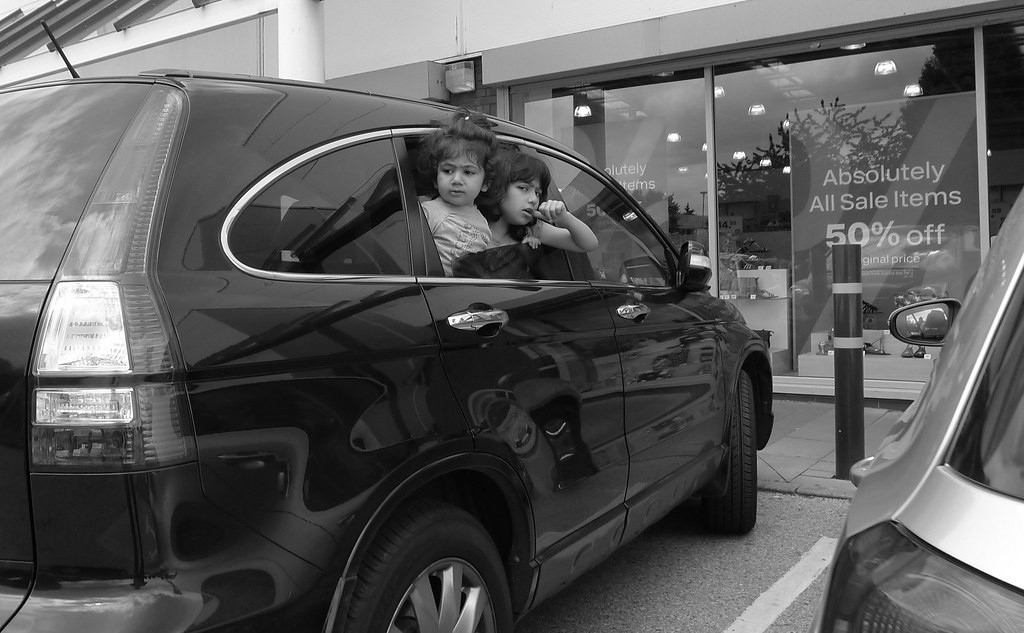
[0,64,777,632]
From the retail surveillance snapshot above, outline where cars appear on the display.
[808,174,1023,632]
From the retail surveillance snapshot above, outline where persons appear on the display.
[485,151,598,257]
[419,108,498,277]
